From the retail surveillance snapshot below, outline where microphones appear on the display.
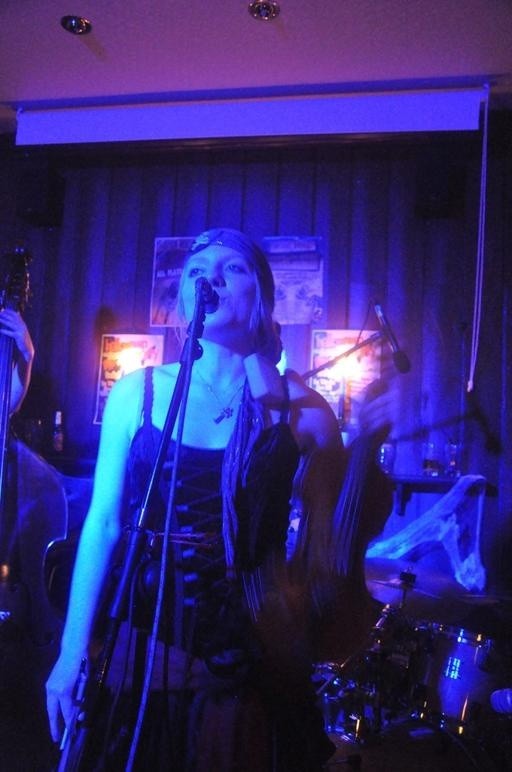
[195,277,219,314]
[466,391,503,457]
[372,297,410,373]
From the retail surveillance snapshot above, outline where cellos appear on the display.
[0,246,69,735]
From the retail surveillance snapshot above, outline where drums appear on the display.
[411,619,498,732]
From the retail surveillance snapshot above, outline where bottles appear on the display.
[49,409,66,462]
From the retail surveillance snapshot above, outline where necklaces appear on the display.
[192,356,247,427]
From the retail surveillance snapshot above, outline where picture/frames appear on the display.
[91,329,168,428]
[302,323,391,434]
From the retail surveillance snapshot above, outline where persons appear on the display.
[1,300,69,650]
[43,224,349,772]
